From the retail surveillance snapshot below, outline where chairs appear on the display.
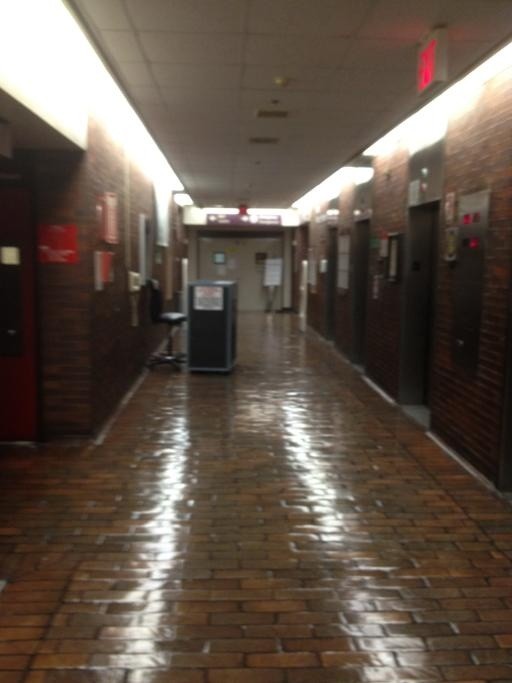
[145,278,188,370]
